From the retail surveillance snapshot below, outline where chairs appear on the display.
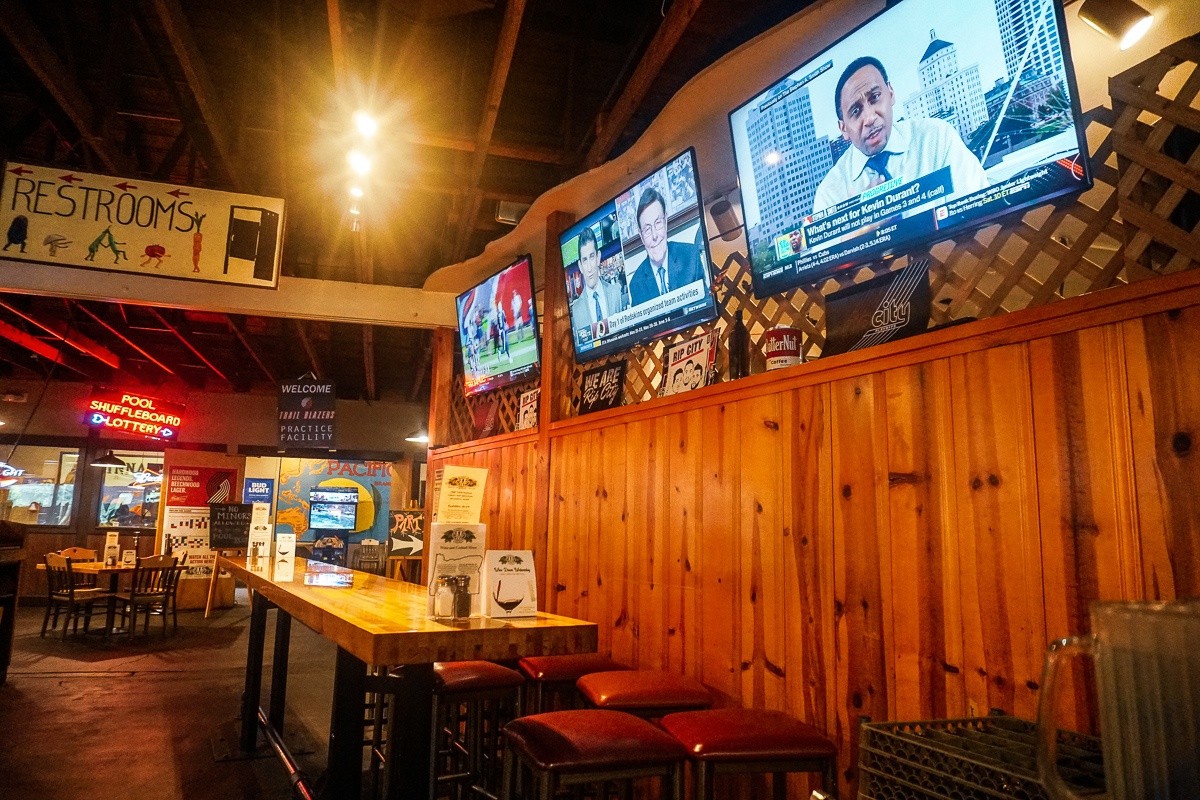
[40,546,189,642]
[358,539,379,574]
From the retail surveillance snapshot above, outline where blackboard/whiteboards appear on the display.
[209,504,252,548]
[388,509,423,560]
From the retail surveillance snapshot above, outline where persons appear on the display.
[465,289,535,377]
[569,227,621,342]
[628,187,708,327]
[812,54,989,256]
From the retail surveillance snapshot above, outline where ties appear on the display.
[593,290,603,322]
[658,267,668,296]
[866,151,894,182]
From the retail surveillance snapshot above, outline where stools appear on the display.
[434,653,838,800]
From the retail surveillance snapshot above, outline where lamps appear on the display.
[90,450,126,467]
[404,421,428,442]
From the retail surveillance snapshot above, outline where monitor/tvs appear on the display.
[308,501,357,531]
[726,0,1091,300]
[455,253,547,398]
[557,145,720,364]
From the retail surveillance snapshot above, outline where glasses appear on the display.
[640,215,666,238]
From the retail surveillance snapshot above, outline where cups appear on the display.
[1035,600,1200,800]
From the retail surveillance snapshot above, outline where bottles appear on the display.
[106,555,116,566]
[257,542,265,558]
[452,575,472,624]
[433,576,455,624]
[250,542,258,558]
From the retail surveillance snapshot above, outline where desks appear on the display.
[35,561,188,639]
[214,554,599,800]
